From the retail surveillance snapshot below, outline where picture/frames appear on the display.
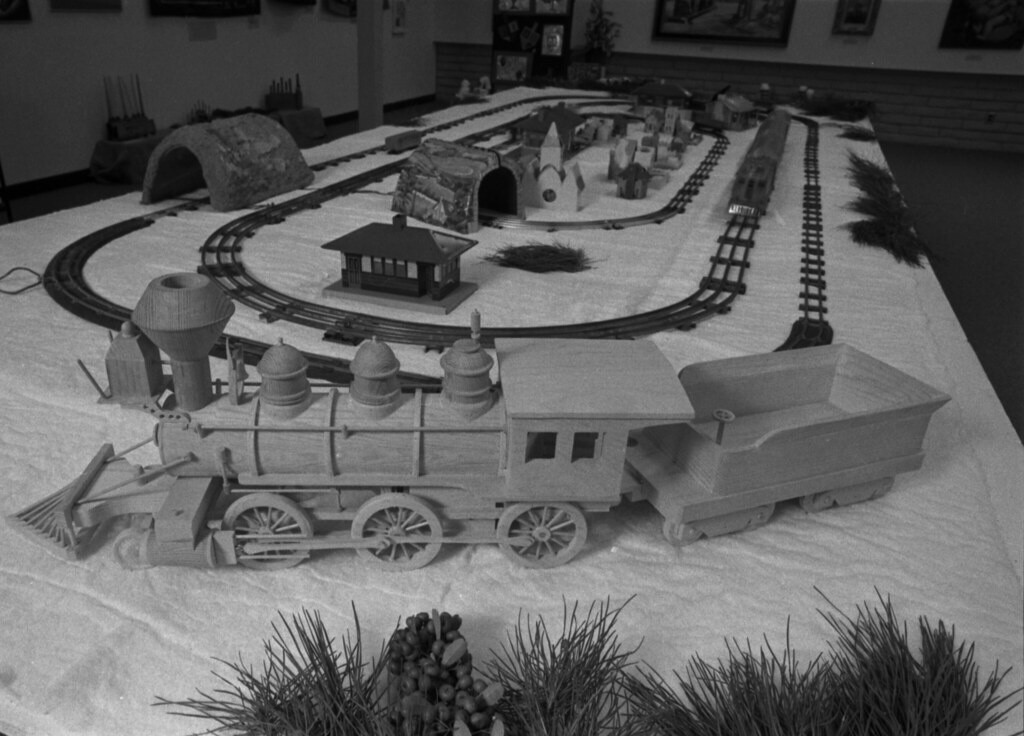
[831,0,881,37]
[937,0,1024,50]
[651,0,797,49]
[492,52,533,86]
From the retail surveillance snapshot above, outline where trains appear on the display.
[726,109,793,219]
[4,269,954,575]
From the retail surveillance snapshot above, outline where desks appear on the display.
[0,83,1024,736]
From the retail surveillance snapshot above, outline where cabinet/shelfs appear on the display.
[493,0,574,77]
